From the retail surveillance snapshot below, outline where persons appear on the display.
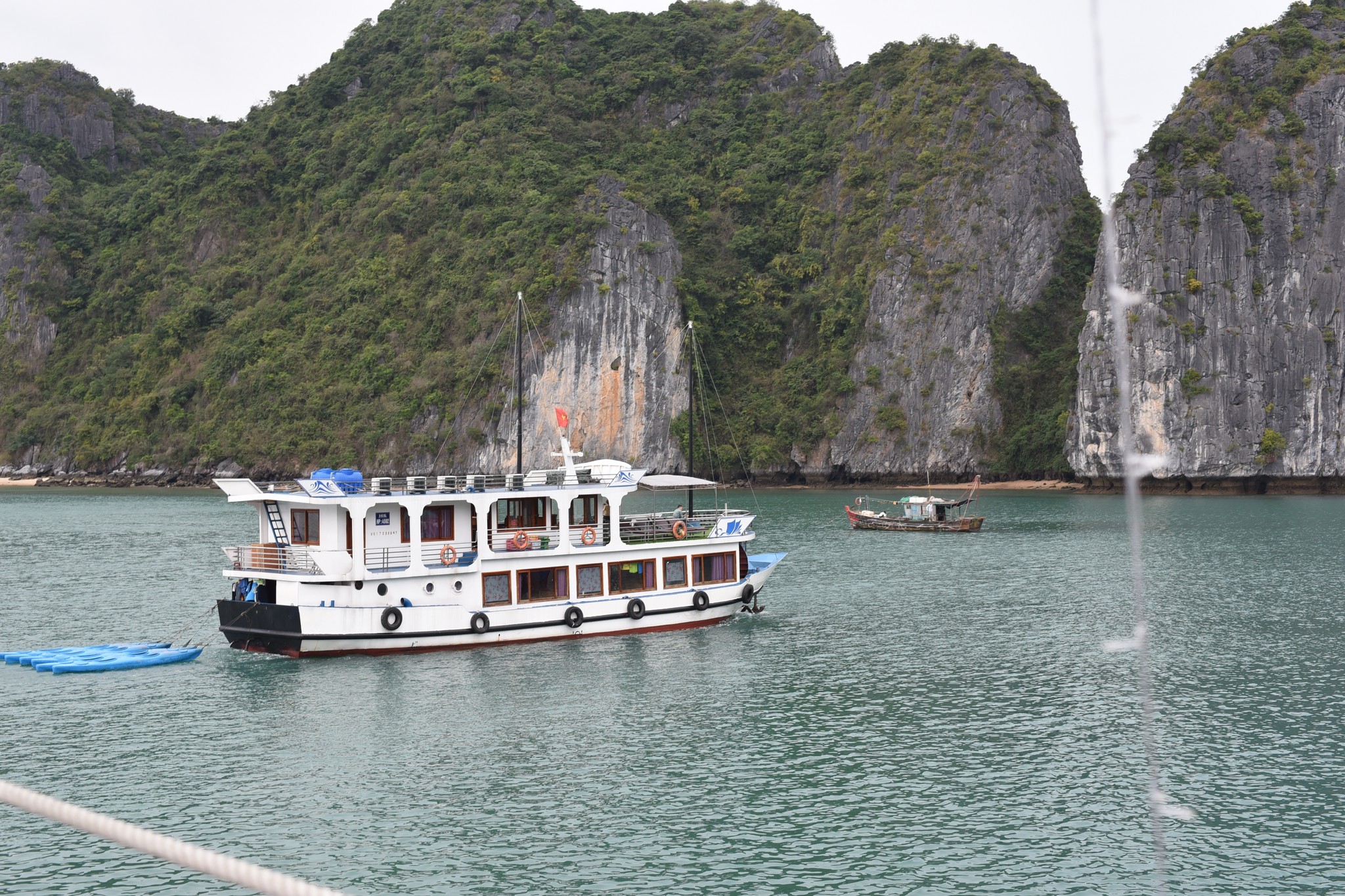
[926,501,935,521]
[673,505,689,527]
[603,499,610,520]
[879,511,886,518]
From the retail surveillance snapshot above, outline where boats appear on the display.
[847,478,991,531]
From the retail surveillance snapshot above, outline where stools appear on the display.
[233,563,241,571]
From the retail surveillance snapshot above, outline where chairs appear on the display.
[620,517,709,542]
[531,514,539,525]
[552,514,557,524]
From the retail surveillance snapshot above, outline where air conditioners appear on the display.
[407,476,427,493]
[466,475,485,491]
[506,474,524,490]
[372,477,392,495]
[437,476,456,491]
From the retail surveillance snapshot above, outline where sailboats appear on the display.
[211,292,789,659]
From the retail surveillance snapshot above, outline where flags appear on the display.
[555,408,569,428]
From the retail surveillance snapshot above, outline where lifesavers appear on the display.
[673,521,687,540]
[582,527,596,545]
[381,607,402,630]
[693,591,709,611]
[470,612,489,634]
[441,546,456,565]
[855,497,862,506]
[564,607,583,628]
[513,531,529,550]
[742,584,753,604]
[627,599,645,620]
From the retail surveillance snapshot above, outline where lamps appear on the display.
[267,485,274,492]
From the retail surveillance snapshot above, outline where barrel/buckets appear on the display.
[688,522,701,527]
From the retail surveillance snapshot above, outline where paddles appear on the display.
[827,505,855,523]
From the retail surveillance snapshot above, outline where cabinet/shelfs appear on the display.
[251,544,286,570]
